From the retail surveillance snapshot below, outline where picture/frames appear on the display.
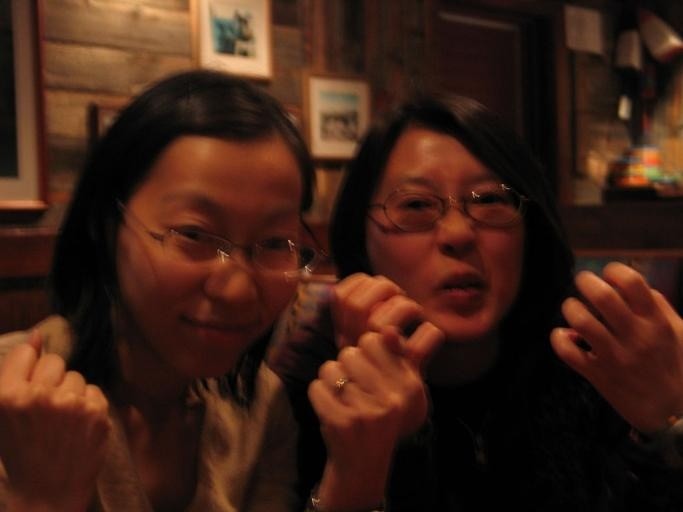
[186,0,275,82]
[300,74,372,163]
[0,0,49,211]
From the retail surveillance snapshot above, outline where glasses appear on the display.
[115,198,327,278]
[370,184,532,232]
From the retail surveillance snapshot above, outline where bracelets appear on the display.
[307,487,387,512]
[629,418,683,453]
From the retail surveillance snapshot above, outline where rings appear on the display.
[334,376,350,400]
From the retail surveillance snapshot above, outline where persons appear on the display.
[0,70,428,512]
[268,89,683,512]
